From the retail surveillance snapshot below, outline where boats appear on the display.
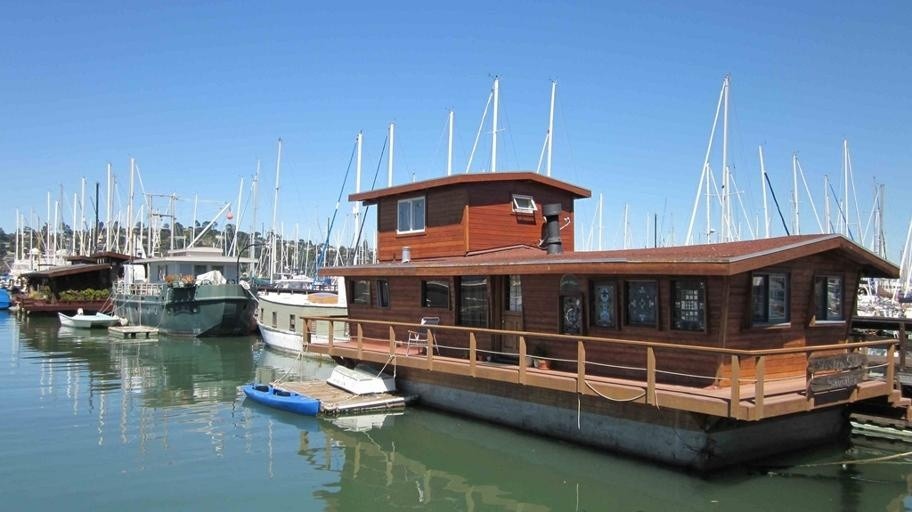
[241,342,408,420]
[299,172,901,473]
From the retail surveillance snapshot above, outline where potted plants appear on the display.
[534,346,553,370]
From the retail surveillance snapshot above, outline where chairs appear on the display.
[408,317,439,355]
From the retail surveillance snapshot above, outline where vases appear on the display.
[533,358,538,368]
[526,353,532,367]
